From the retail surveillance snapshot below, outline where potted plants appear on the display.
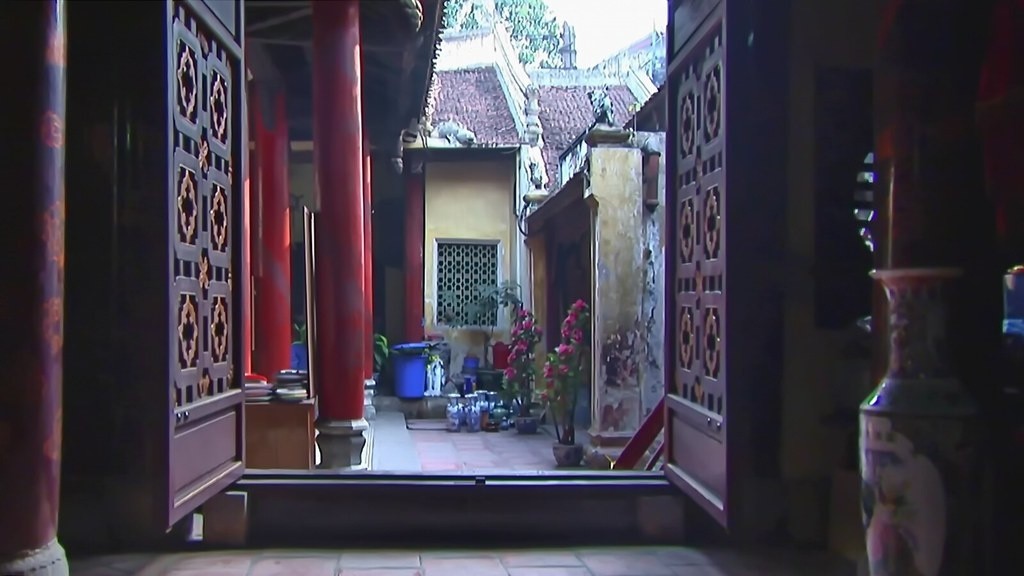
[443,282,524,390]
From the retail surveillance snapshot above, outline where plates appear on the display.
[246,384,309,402]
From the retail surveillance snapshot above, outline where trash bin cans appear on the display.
[391,342,430,399]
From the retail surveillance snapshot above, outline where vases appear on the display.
[448,389,585,465]
[854,266,968,576]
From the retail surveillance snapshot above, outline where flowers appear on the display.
[499,299,589,446]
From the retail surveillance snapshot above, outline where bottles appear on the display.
[445,389,498,433]
[858,263,981,574]
